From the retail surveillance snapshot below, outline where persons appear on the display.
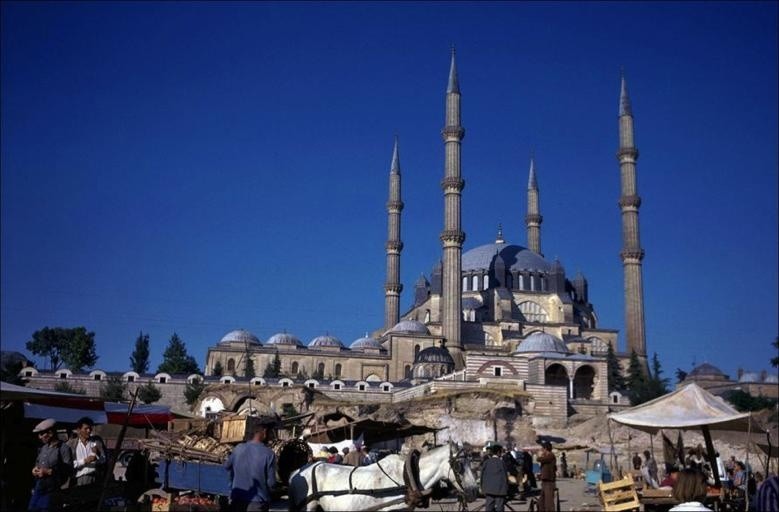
[631,443,778,512]
[60,416,107,511]
[479,444,509,511]
[536,441,558,512]
[479,446,493,467]
[224,419,277,511]
[316,443,375,466]
[30,416,75,512]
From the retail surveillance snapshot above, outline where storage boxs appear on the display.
[220,415,254,444]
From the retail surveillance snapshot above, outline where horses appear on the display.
[288,440,479,512]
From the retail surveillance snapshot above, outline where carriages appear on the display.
[152,432,480,512]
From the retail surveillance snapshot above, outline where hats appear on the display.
[32,418,56,433]
[256,416,282,430]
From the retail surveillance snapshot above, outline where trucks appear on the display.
[104,435,166,467]
